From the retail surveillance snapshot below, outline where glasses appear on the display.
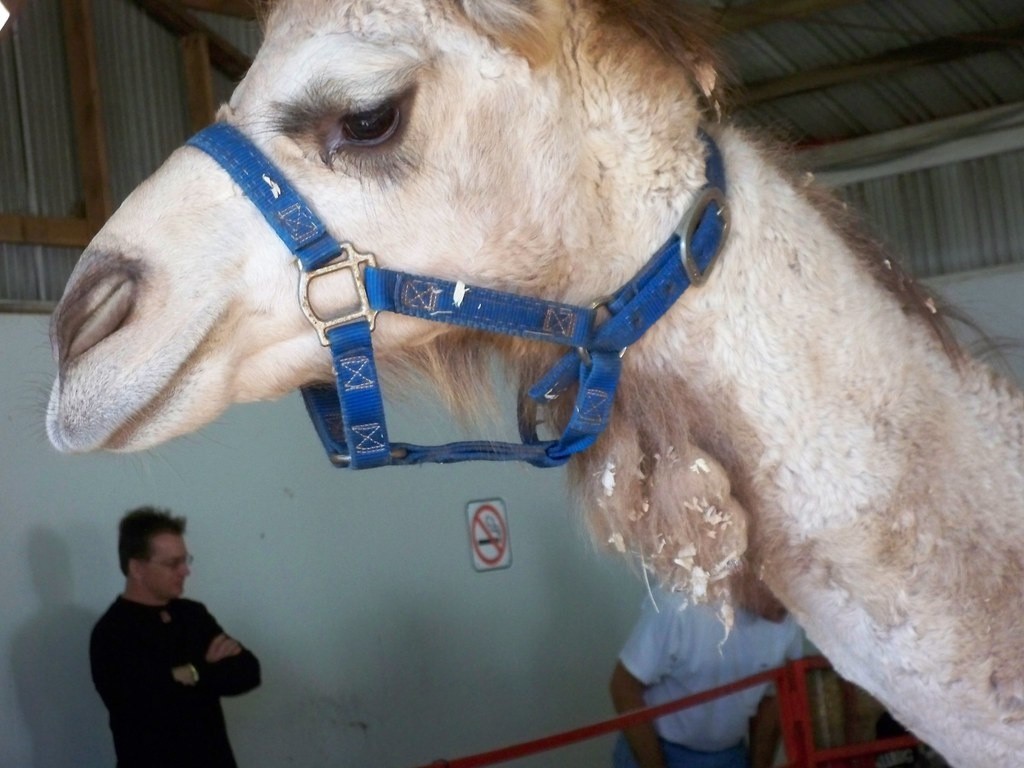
[141,553,194,571]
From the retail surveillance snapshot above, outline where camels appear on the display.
[11,0,1024,768]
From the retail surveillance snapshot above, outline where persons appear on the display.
[89,506,262,768]
[608,573,808,768]
